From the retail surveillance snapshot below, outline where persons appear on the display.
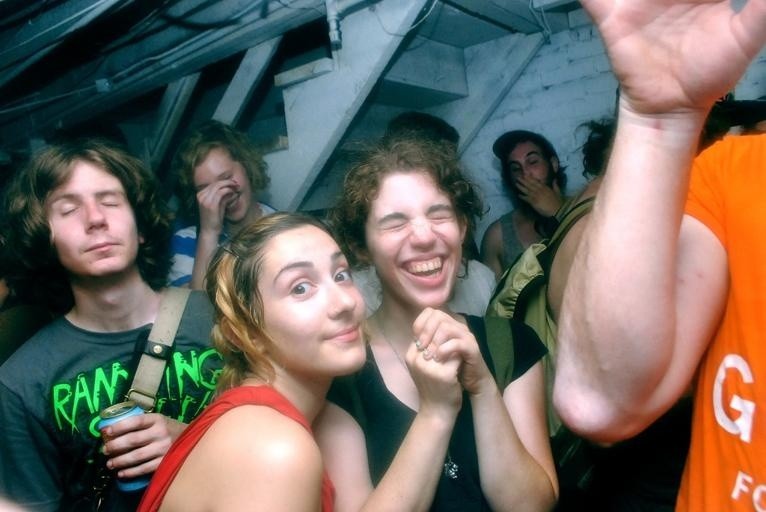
[0,125,248,512]
[480,131,578,281]
[164,118,283,291]
[136,208,373,512]
[542,86,739,510]
[549,1,766,510]
[347,108,500,323]
[310,131,562,510]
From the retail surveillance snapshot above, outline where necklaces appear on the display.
[374,308,462,481]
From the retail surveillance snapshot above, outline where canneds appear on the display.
[98,401,150,492]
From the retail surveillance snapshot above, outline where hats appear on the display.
[493,131,556,164]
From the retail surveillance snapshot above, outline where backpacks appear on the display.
[484,191,596,443]
